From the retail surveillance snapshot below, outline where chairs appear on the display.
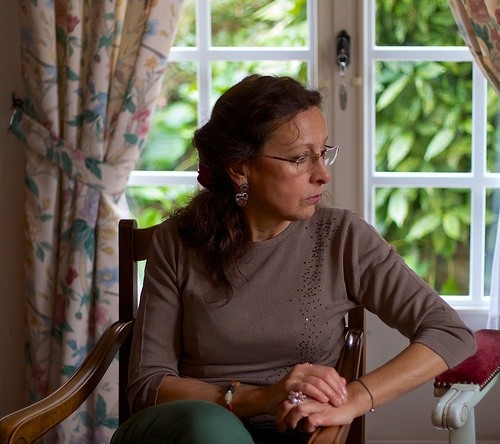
[0,219,366,444]
[431,218,500,444]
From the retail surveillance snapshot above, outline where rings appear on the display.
[287,389,306,406]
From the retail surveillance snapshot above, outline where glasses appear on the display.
[255,144,340,174]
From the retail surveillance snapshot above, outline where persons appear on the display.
[109,74,478,444]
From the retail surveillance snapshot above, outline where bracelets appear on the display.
[224,380,240,411]
[354,379,375,413]
